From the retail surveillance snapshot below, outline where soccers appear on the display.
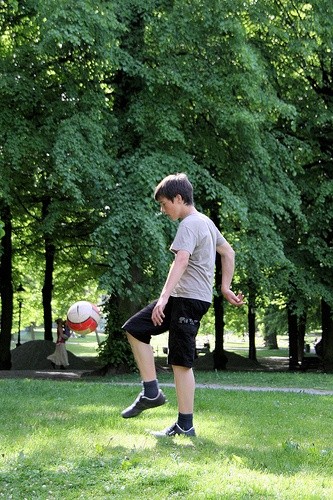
[67,301,100,334]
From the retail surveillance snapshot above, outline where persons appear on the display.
[62,319,74,340]
[46,318,71,369]
[121,172,246,439]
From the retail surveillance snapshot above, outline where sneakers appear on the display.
[150,422,196,439]
[121,389,167,418]
